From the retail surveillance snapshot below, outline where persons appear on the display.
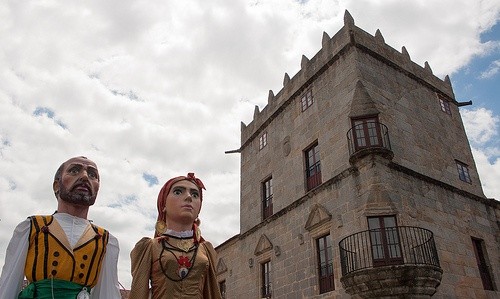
[127,172,224,299]
[0,156,123,299]
